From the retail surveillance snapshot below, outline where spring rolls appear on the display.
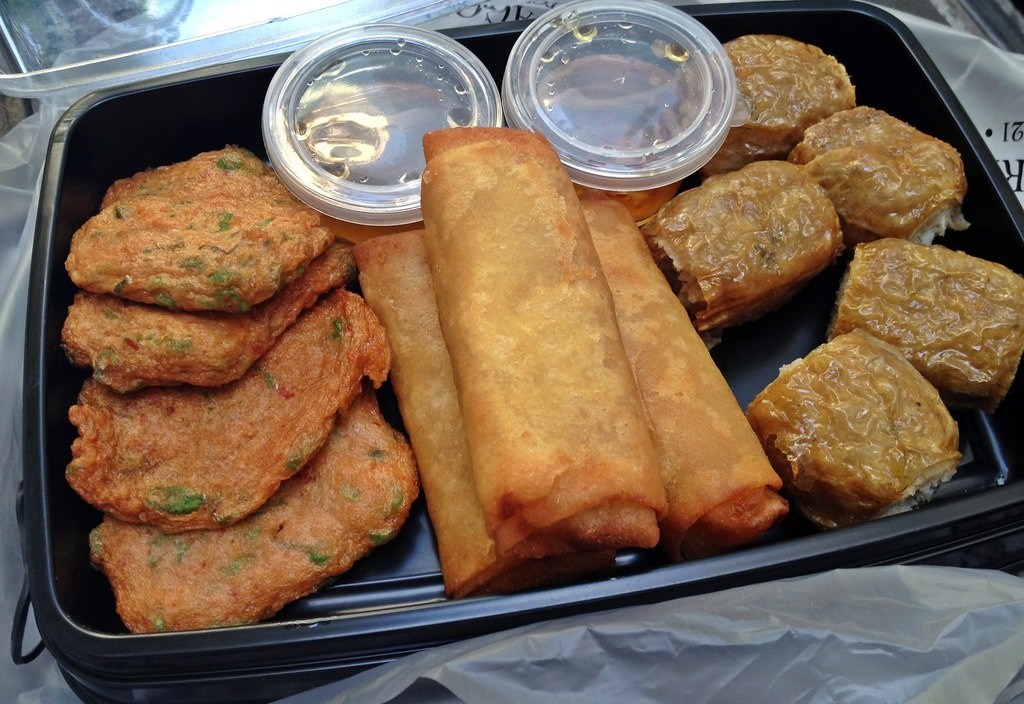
[349,124,787,600]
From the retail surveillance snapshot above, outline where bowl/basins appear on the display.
[259,21,504,246]
[498,0,752,224]
